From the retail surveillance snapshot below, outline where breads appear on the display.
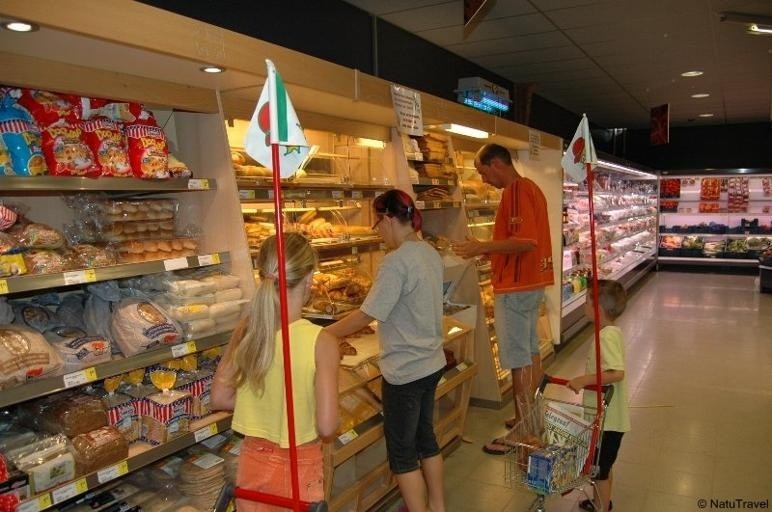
[0,391,129,504]
[53,479,201,512]
[132,429,245,512]
[304,268,374,314]
[243,211,334,248]
[231,149,272,177]
[123,266,248,341]
[0,281,183,388]
[82,348,223,446]
[0,198,199,280]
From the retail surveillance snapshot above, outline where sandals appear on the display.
[579,500,612,512]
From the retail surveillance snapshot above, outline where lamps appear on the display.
[458,97,495,114]
[744,24,772,36]
[476,97,508,112]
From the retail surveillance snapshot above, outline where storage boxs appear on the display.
[230,144,274,180]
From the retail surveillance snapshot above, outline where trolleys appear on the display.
[487,364,616,512]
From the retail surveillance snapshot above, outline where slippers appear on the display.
[482,439,515,454]
[506,419,516,428]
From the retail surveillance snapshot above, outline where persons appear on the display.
[210,232,342,512]
[451,143,556,456]
[323,188,448,512]
[568,277,629,512]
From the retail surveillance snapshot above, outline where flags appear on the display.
[560,113,597,185]
[242,60,310,179]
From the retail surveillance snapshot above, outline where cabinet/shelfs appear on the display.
[561,142,659,344]
[215,25,501,511]
[449,106,561,411]
[0,0,257,512]
[657,168,772,268]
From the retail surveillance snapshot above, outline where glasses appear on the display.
[372,219,383,232]
